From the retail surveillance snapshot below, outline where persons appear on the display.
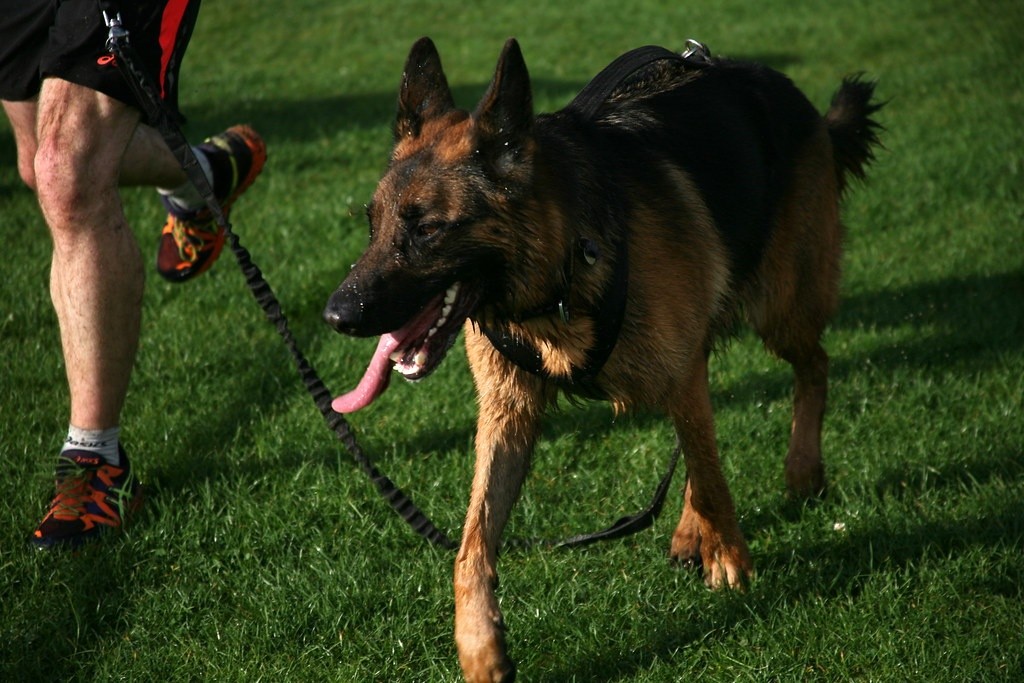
[1,1,269,553]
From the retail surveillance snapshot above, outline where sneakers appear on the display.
[35,445,140,543]
[157,126,268,283]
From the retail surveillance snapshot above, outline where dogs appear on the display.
[322,35,894,683]
